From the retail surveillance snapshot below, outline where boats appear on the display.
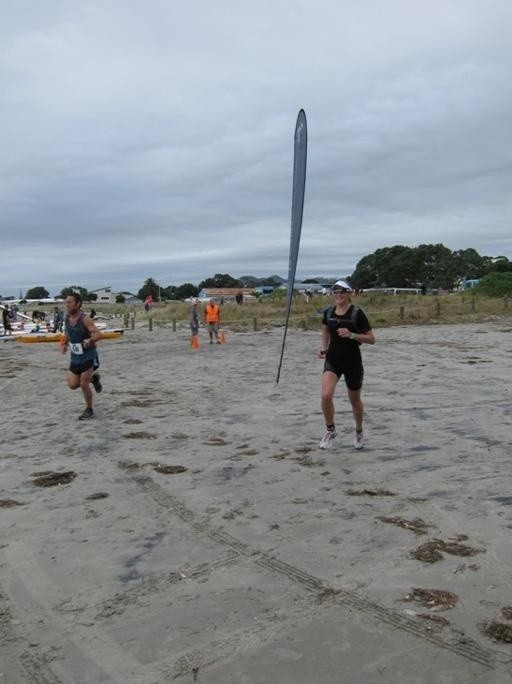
[0,321,124,343]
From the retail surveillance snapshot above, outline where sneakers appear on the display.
[319,427,338,449]
[79,407,95,420]
[353,424,365,449]
[92,372,103,392]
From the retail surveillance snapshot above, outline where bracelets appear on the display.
[350,333,357,340]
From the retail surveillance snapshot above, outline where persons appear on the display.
[144,295,153,311]
[236,291,243,305]
[190,303,199,335]
[60,292,102,421]
[90,310,96,318]
[204,300,221,344]
[2,304,63,336]
[319,280,376,450]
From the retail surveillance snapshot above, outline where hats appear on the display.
[331,279,353,292]
